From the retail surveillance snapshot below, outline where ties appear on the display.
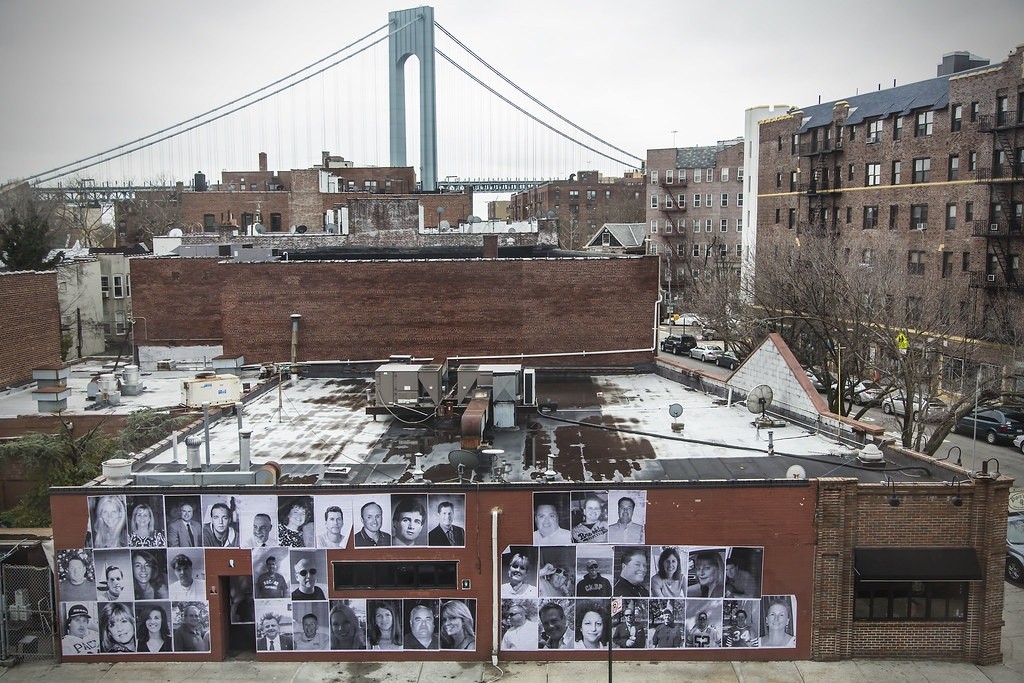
[187,524,194,547]
[270,642,274,650]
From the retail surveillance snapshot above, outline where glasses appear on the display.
[625,614,633,617]
[294,569,317,576]
[556,568,568,577]
[590,565,598,569]
[508,612,520,617]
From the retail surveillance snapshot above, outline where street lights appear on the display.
[834,343,846,443]
[644,239,671,304]
[283,252,288,261]
[971,363,1001,472]
[462,203,468,233]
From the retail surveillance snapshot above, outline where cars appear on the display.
[804,369,838,389]
[949,403,1024,444]
[667,313,708,326]
[715,351,747,370]
[661,334,697,355]
[881,392,949,424]
[1013,435,1024,454]
[689,344,723,361]
[831,378,884,405]
[1005,512,1024,583]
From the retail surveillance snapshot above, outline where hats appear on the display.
[586,560,598,567]
[69,605,91,618]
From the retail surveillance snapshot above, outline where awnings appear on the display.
[854,547,983,582]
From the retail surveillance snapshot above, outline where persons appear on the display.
[58,494,239,654]
[501,496,796,650]
[241,502,476,651]
